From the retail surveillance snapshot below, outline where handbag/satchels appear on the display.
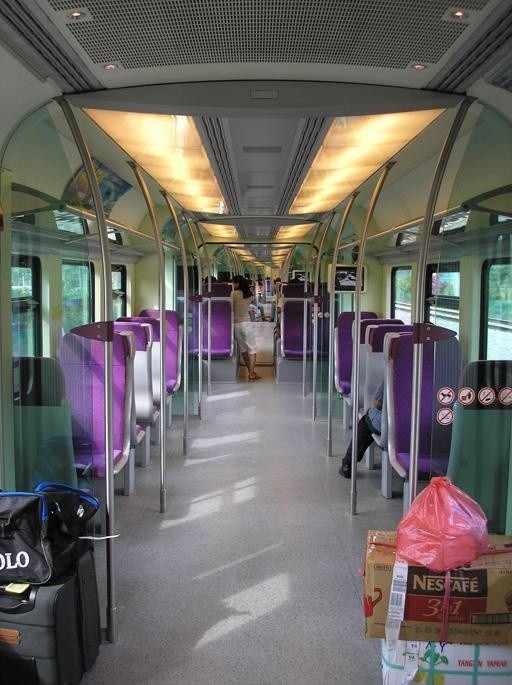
[1,482,101,586]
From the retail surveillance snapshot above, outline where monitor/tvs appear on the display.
[328,264,367,293]
[292,270,311,283]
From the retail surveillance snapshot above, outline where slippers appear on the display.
[248,372,261,379]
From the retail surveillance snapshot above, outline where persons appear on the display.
[339,377,384,478]
[202,275,281,380]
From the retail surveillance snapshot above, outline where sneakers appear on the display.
[339,464,351,478]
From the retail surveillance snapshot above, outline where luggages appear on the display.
[0,551,102,685]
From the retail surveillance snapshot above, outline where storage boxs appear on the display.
[364,528,512,685]
